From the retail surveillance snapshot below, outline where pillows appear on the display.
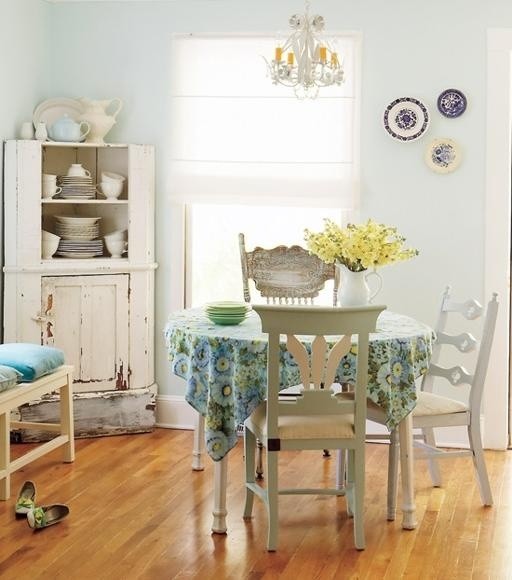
[0,344,64,392]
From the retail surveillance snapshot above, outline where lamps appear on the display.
[263,1,347,100]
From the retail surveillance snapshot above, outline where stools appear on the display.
[0,366,75,501]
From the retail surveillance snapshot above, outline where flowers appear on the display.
[304,218,420,272]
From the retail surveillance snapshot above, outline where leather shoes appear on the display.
[15,480,36,516]
[27,504,69,528]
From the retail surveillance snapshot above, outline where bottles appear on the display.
[20,122,34,139]
[76,98,122,144]
[35,122,47,140]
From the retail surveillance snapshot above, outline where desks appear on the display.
[168,306,434,536]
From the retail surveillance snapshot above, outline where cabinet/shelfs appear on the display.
[4,139,156,443]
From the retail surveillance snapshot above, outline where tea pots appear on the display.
[48,113,91,142]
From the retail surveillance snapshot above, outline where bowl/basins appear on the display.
[104,229,129,258]
[42,174,62,200]
[101,171,125,182]
[95,181,124,200]
[42,229,62,259]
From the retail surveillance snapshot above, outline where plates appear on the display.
[52,215,105,258]
[32,98,85,132]
[383,96,431,143]
[204,300,253,326]
[59,175,96,199]
[426,137,462,174]
[436,87,468,119]
